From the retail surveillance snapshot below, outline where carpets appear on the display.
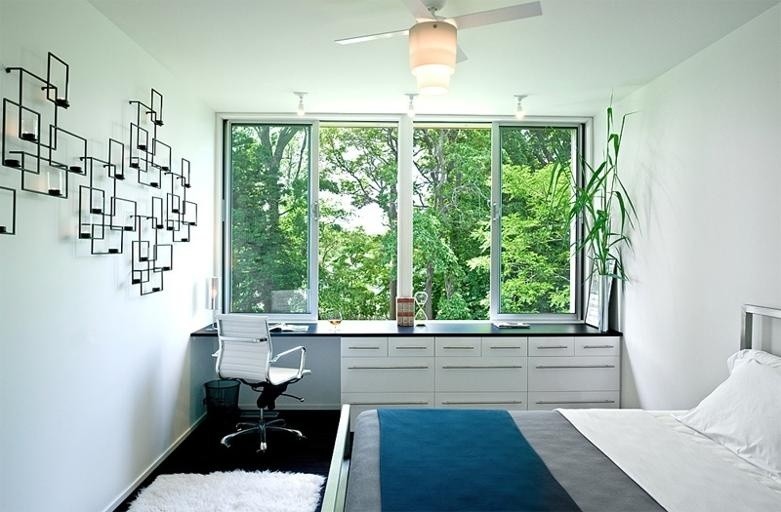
[126,468,327,512]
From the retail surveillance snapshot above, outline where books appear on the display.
[491,320,531,329]
[268,322,310,332]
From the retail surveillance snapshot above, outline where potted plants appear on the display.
[545,87,642,333]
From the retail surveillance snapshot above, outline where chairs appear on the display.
[211,311,313,453]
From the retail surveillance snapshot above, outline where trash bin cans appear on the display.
[204,379,241,426]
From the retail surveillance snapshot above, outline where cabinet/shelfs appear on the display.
[340,336,622,433]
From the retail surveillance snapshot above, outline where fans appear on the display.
[332,0,543,64]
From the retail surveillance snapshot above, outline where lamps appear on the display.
[512,94,528,123]
[406,22,458,96]
[294,91,309,117]
[405,92,418,119]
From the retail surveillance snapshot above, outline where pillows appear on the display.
[670,348,781,483]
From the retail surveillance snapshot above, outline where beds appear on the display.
[320,303,781,512]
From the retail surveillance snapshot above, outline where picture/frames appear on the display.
[585,257,618,329]
